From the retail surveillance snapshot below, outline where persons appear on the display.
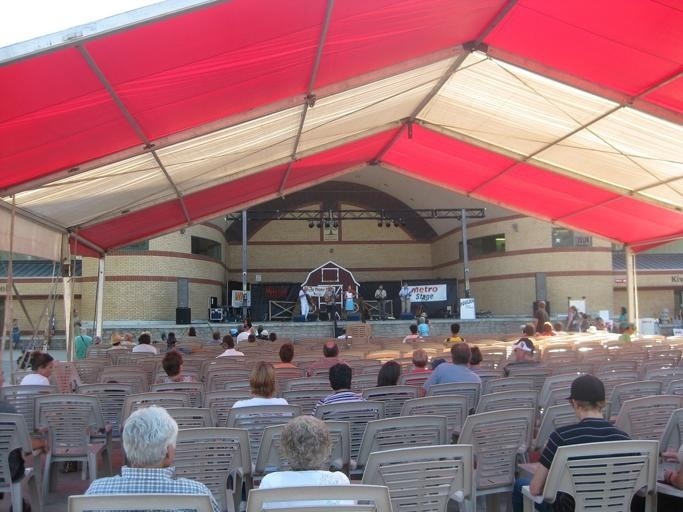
[257,416,355,508]
[231,361,292,459]
[0,308,202,411]
[511,375,642,508]
[503,297,643,367]
[312,363,375,459]
[0,372,53,480]
[208,281,485,415]
[661,440,683,490]
[84,405,220,507]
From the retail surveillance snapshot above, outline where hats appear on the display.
[520,323,534,336]
[565,375,605,401]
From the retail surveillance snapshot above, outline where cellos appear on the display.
[416,296,423,316]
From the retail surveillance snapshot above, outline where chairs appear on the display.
[0,316,682,512]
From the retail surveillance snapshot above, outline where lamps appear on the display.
[223,208,486,231]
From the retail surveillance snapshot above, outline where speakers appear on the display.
[176,307,191,324]
[534,301,550,317]
